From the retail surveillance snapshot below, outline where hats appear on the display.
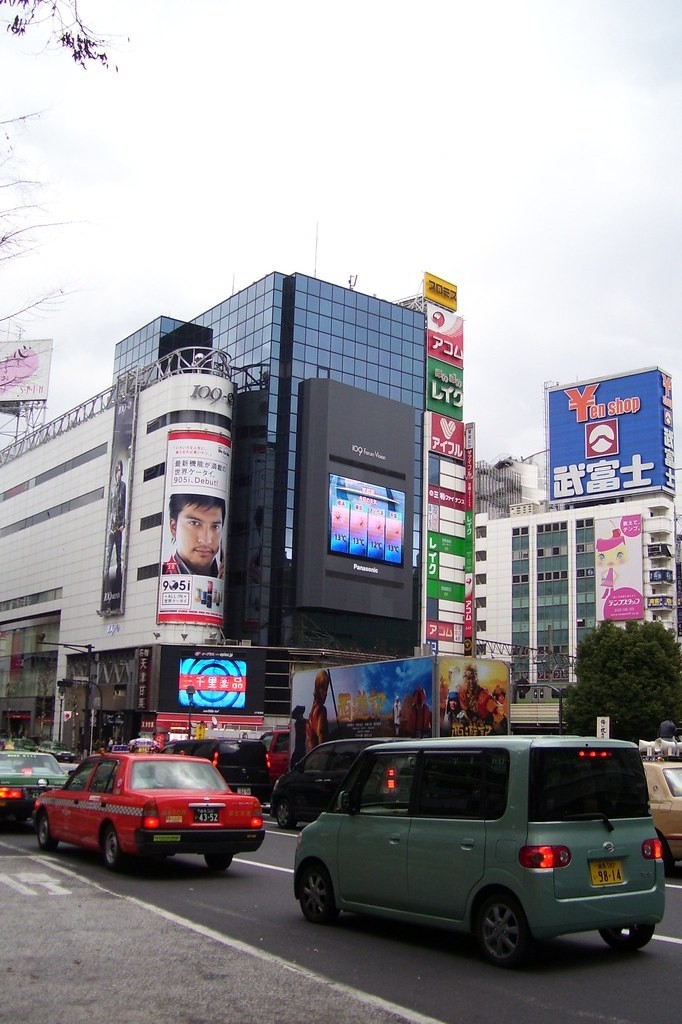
[446,692,461,715]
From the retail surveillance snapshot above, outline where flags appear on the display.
[64,711,71,722]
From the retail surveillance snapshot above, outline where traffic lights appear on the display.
[534,689,537,698]
[540,688,544,698]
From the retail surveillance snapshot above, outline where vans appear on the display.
[158,738,273,805]
[269,736,419,830]
[294,736,664,971]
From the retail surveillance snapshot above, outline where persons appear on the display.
[392,696,402,736]
[162,494,226,581]
[307,671,330,752]
[104,460,126,580]
[407,687,430,738]
[443,665,508,730]
[291,705,309,767]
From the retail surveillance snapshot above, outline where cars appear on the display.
[32,745,265,875]
[0,749,82,835]
[35,741,75,762]
[638,738,682,878]
[0,738,38,753]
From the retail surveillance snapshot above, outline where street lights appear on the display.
[186,686,195,739]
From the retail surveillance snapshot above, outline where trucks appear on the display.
[259,654,511,791]
